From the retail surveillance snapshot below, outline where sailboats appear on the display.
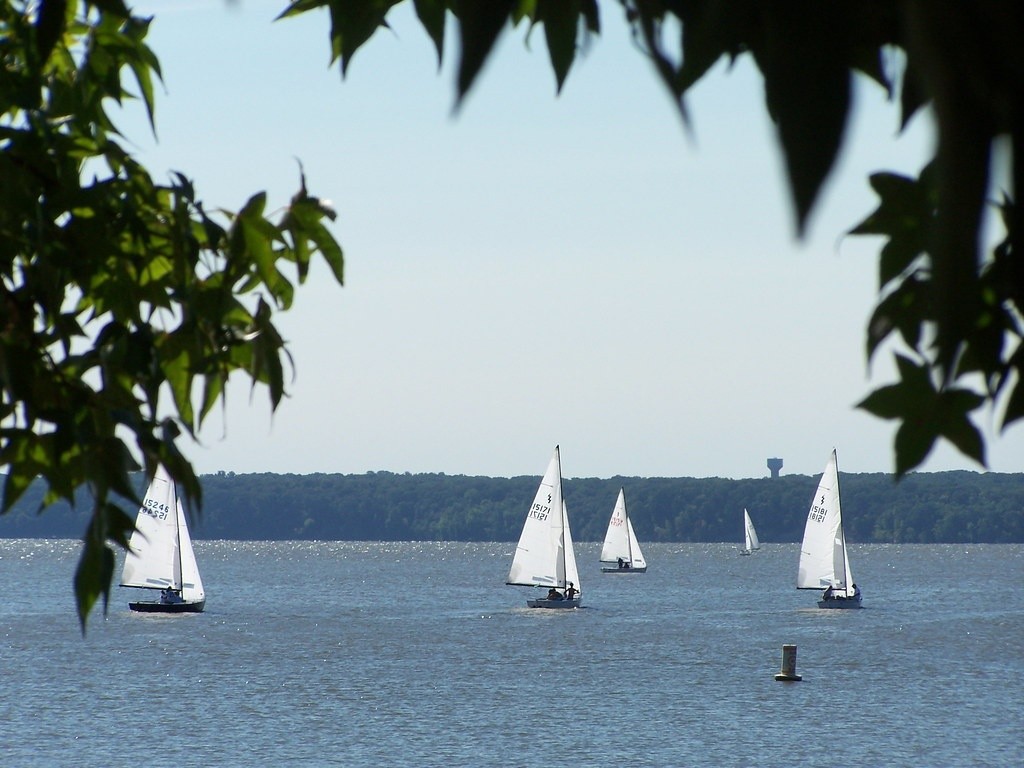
[796,444,864,609]
[597,486,649,574]
[506,444,584,610]
[118,461,206,613]
[739,507,762,556]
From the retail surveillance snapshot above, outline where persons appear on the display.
[548,582,579,600]
[618,558,629,568]
[823,585,836,601]
[852,584,860,601]
